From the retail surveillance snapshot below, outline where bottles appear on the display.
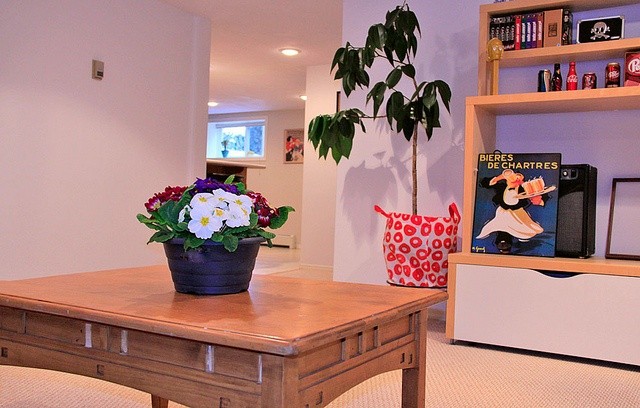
[552,63,562,91]
[566,61,577,90]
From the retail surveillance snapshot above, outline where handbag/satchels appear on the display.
[374,203,461,289]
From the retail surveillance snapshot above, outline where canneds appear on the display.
[581,73,597,90]
[604,62,620,89]
[537,69,551,92]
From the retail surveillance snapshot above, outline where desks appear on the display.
[0,263,450,408]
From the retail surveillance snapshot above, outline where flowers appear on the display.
[132,173,299,256]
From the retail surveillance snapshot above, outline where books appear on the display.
[471,153,562,258]
[489,9,573,51]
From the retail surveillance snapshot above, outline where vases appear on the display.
[162,237,265,298]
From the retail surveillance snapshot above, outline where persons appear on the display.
[286,136,295,161]
[475,169,550,254]
[291,139,303,163]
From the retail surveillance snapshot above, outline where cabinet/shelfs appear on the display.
[442,1,640,371]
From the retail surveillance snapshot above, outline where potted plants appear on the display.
[305,0,462,290]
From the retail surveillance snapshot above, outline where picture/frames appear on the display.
[281,127,304,165]
[602,177,640,260]
